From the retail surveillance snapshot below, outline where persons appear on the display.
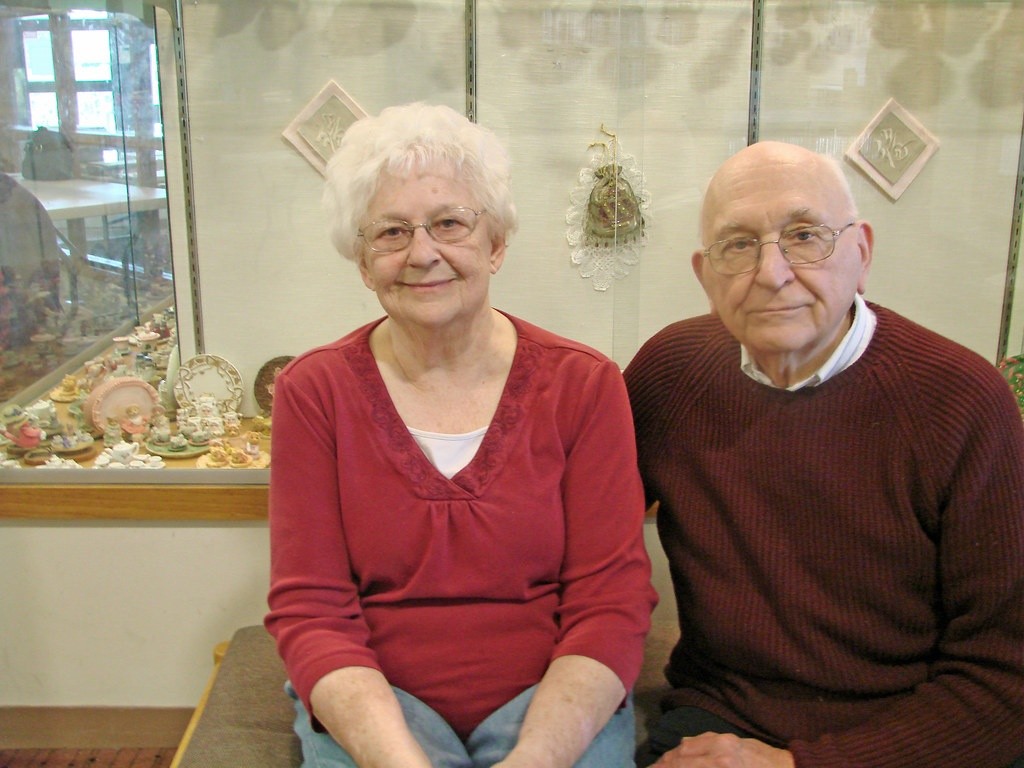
[623,142,1024,768]
[0,171,60,353]
[264,104,660,768]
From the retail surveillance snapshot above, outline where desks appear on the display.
[5,171,166,333]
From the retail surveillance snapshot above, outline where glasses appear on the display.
[357,203,487,253]
[705,222,852,275]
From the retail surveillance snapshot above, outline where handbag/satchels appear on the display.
[22,128,73,179]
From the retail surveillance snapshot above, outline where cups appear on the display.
[137,327,160,352]
[113,337,129,352]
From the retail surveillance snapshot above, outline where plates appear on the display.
[146,438,211,459]
[254,355,297,416]
[173,354,244,411]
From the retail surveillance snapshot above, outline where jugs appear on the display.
[25,399,55,419]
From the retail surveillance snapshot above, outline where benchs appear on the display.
[166,623,682,768]
